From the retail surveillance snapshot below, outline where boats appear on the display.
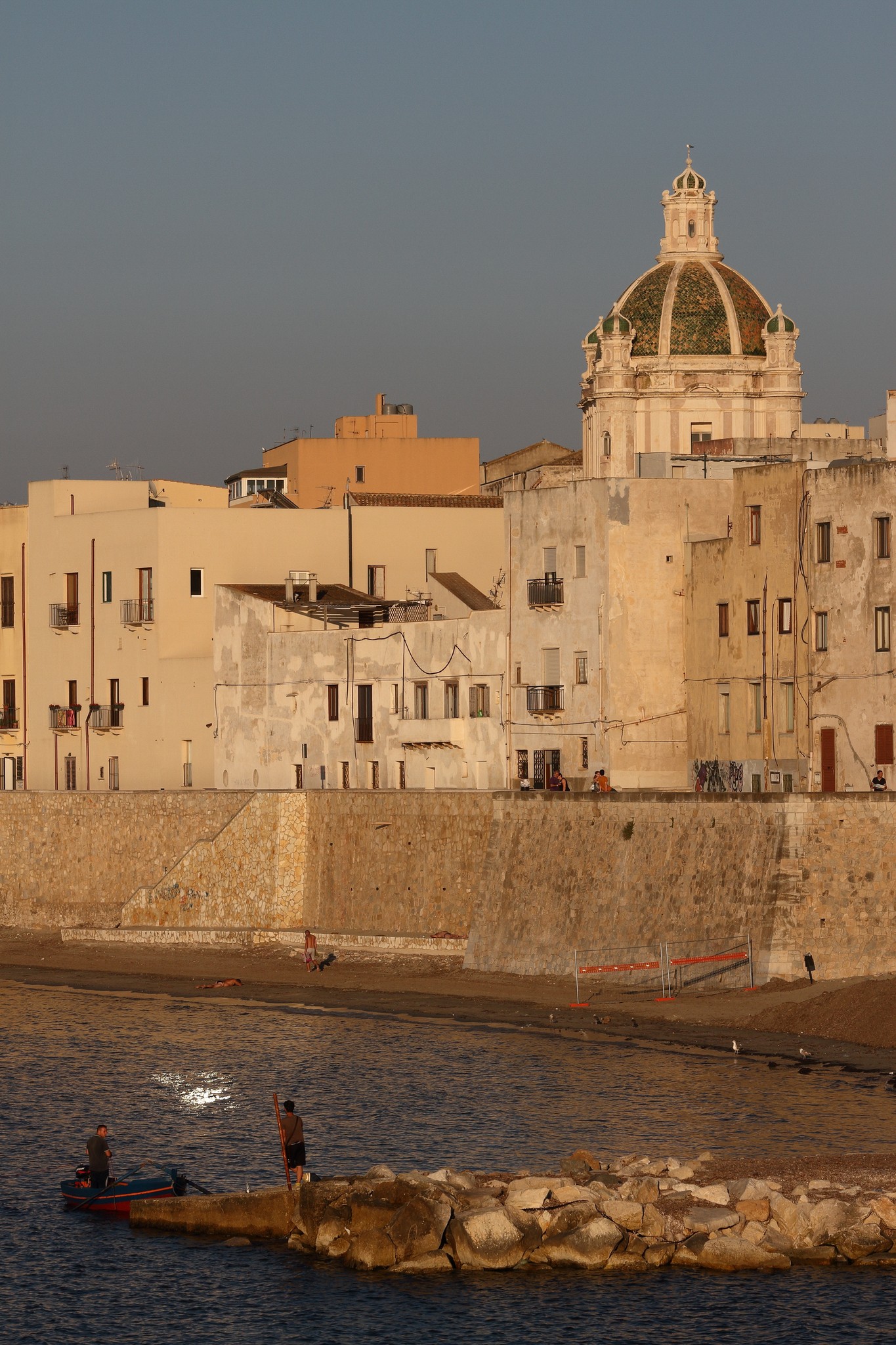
[60,1163,185,1213]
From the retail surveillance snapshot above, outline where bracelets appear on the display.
[556,784,558,787]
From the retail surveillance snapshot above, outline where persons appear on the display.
[277,1100,310,1190]
[593,770,603,792]
[195,979,244,990]
[870,770,887,792]
[596,769,617,793]
[430,931,467,939]
[304,930,321,972]
[549,770,562,791]
[557,772,570,791]
[85,1125,113,1189]
